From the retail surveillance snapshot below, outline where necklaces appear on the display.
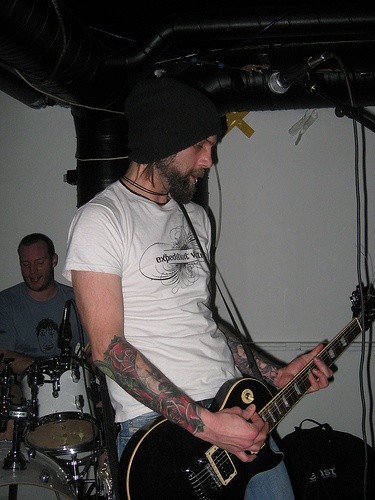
[125,173,169,200]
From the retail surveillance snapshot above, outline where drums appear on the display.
[0,439,78,500]
[19,355,102,453]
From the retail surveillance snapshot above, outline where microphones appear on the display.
[56,302,71,349]
[268,53,333,95]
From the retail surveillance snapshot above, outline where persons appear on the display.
[62,81,336,500]
[0,233,96,451]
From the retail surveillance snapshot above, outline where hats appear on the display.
[125,78,223,164]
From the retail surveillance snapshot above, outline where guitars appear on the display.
[116,283,375,500]
